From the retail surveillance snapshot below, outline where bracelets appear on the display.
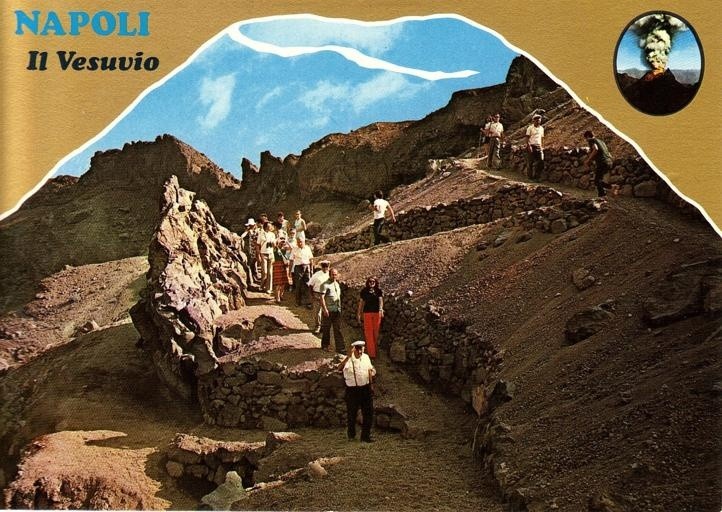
[370,382,373,384]
[378,306,382,310]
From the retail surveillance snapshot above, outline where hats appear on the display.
[244,218,256,226]
[351,340,366,346]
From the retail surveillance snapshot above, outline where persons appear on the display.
[306,260,330,334]
[480,113,506,171]
[241,207,315,309]
[338,340,374,442]
[367,189,396,245]
[582,130,619,200]
[319,268,346,354]
[525,114,546,182]
[356,276,385,360]
